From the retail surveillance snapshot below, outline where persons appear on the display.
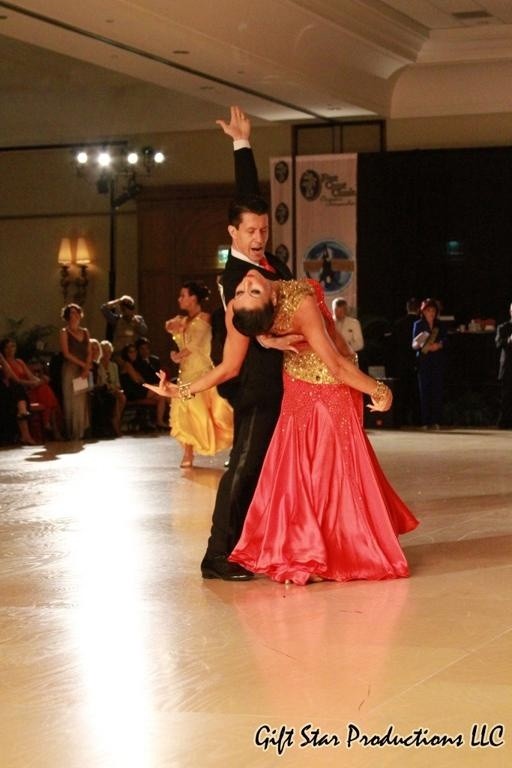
[317,244,340,287]
[495,302,512,429]
[141,267,419,584]
[165,282,236,468]
[209,271,231,467]
[200,103,293,582]
[388,297,420,428]
[0,293,169,445]
[412,298,448,371]
[332,297,364,357]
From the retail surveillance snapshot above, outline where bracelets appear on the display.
[374,380,387,399]
[178,382,194,402]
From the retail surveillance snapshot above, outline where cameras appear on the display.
[124,302,135,310]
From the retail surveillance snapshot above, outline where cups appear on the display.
[459,318,496,333]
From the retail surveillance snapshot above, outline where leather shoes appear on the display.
[223,458,230,467]
[200,553,255,581]
[181,455,194,468]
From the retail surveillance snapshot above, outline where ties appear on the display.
[259,257,277,273]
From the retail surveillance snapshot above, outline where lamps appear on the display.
[57,237,91,301]
[75,145,166,180]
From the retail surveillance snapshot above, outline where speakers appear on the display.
[366,378,400,428]
